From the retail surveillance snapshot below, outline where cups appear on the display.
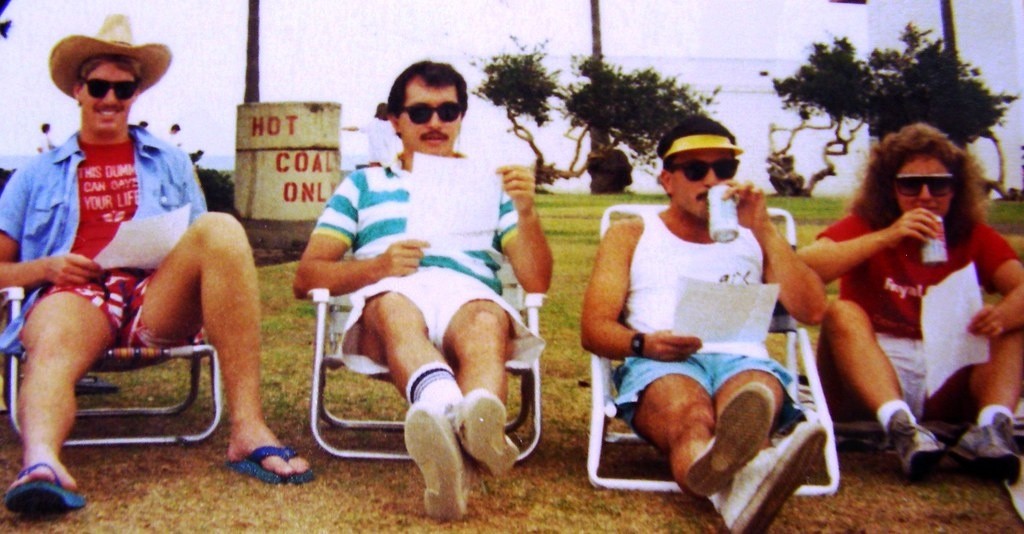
[921,216,948,265]
[708,184,739,243]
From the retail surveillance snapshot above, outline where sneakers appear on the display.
[887,409,944,478]
[951,411,1021,486]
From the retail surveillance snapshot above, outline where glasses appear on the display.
[891,175,957,197]
[394,102,464,124]
[83,76,139,101]
[665,158,739,180]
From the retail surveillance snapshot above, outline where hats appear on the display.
[49,14,173,99]
[662,133,744,159]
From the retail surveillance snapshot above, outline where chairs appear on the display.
[306,284,548,462]
[0,284,222,446]
[588,205,841,496]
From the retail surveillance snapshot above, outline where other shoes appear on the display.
[455,389,520,477]
[404,401,468,520]
[685,383,775,497]
[696,422,826,534]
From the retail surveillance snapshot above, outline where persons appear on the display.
[0,13,314,515]
[579,114,827,534]
[35,123,54,153]
[292,60,554,520]
[341,103,397,163]
[170,124,186,153]
[795,121,1023,488]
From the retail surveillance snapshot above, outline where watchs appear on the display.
[631,333,646,359]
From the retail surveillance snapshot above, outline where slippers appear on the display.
[226,445,313,484]
[3,463,86,517]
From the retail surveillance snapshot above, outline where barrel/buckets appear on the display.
[234,101,342,246]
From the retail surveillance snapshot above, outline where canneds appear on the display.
[708,185,738,242]
[918,215,948,264]
